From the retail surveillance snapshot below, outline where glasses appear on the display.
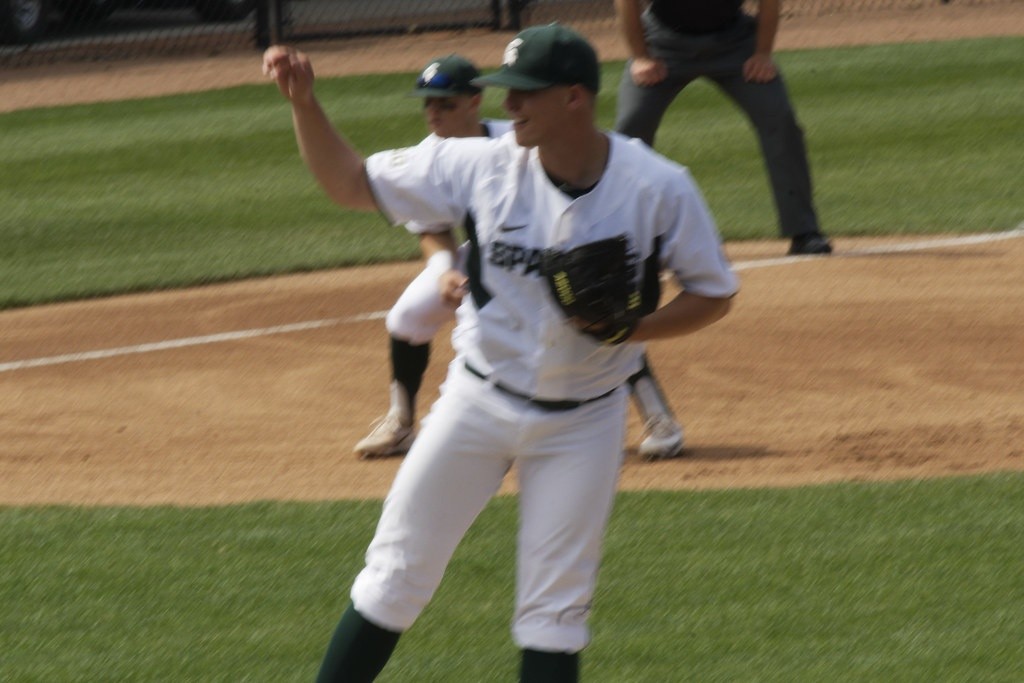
[414,73,467,90]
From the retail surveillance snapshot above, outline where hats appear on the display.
[470,21,600,92]
[406,54,482,96]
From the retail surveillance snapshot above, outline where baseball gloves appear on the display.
[544,231,642,344]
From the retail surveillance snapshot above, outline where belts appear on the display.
[465,363,620,412]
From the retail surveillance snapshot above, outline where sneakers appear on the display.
[353,412,416,456]
[789,233,833,253]
[636,415,685,458]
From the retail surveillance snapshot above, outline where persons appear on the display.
[261,22,739,683]
[352,54,685,459]
[613,0,831,252]
